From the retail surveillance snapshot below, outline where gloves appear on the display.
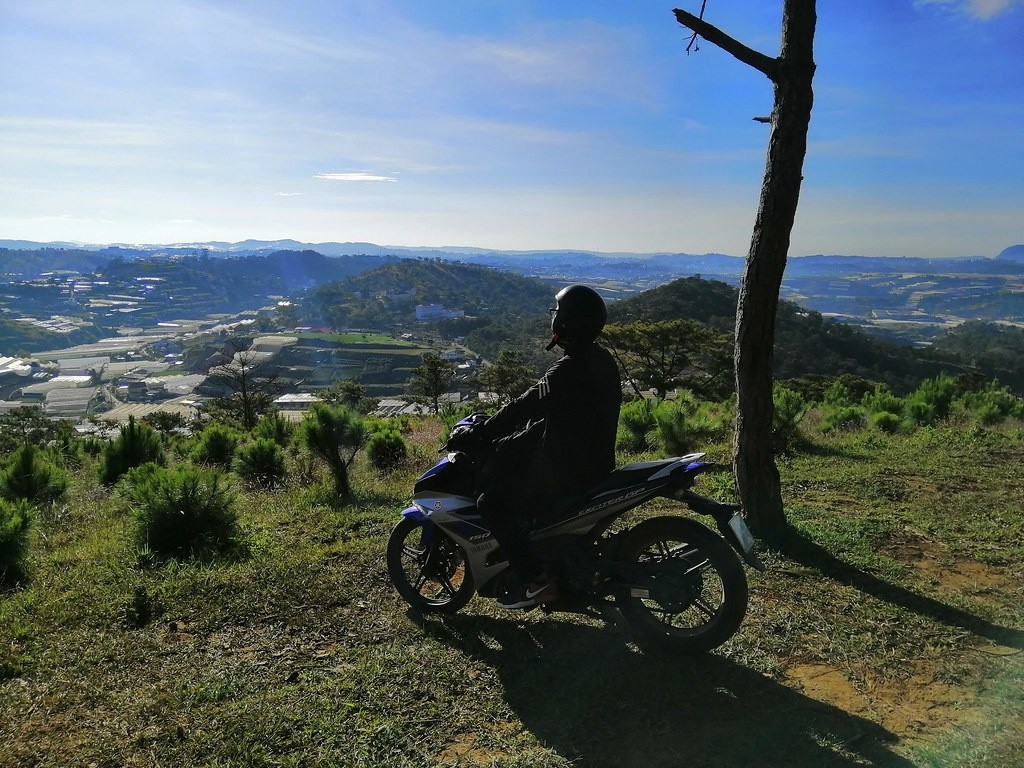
[447,419,487,451]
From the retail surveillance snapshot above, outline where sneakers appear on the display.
[496,582,558,608]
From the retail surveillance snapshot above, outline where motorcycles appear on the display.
[386,411,767,653]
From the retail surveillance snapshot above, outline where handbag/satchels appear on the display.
[484,417,548,481]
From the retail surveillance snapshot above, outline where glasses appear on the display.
[549,308,558,316]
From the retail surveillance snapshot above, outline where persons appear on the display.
[446,284,622,609]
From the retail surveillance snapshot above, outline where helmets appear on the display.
[556,284,608,337]
